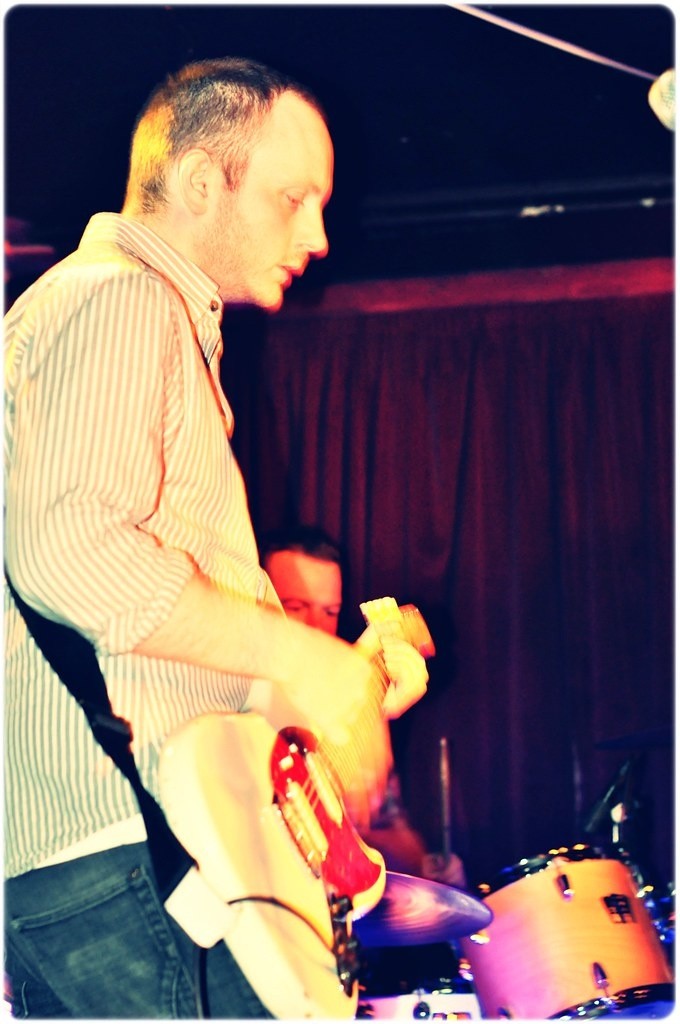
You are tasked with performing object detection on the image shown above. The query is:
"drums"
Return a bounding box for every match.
[458,841,676,1020]
[356,975,482,1021]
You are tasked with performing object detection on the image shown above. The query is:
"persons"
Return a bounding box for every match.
[260,526,469,992]
[3,55,430,1020]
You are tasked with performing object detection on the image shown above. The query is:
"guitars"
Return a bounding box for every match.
[154,592,438,1021]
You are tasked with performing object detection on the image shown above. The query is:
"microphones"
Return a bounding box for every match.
[586,762,632,835]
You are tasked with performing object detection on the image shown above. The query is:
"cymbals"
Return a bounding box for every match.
[348,869,496,951]
